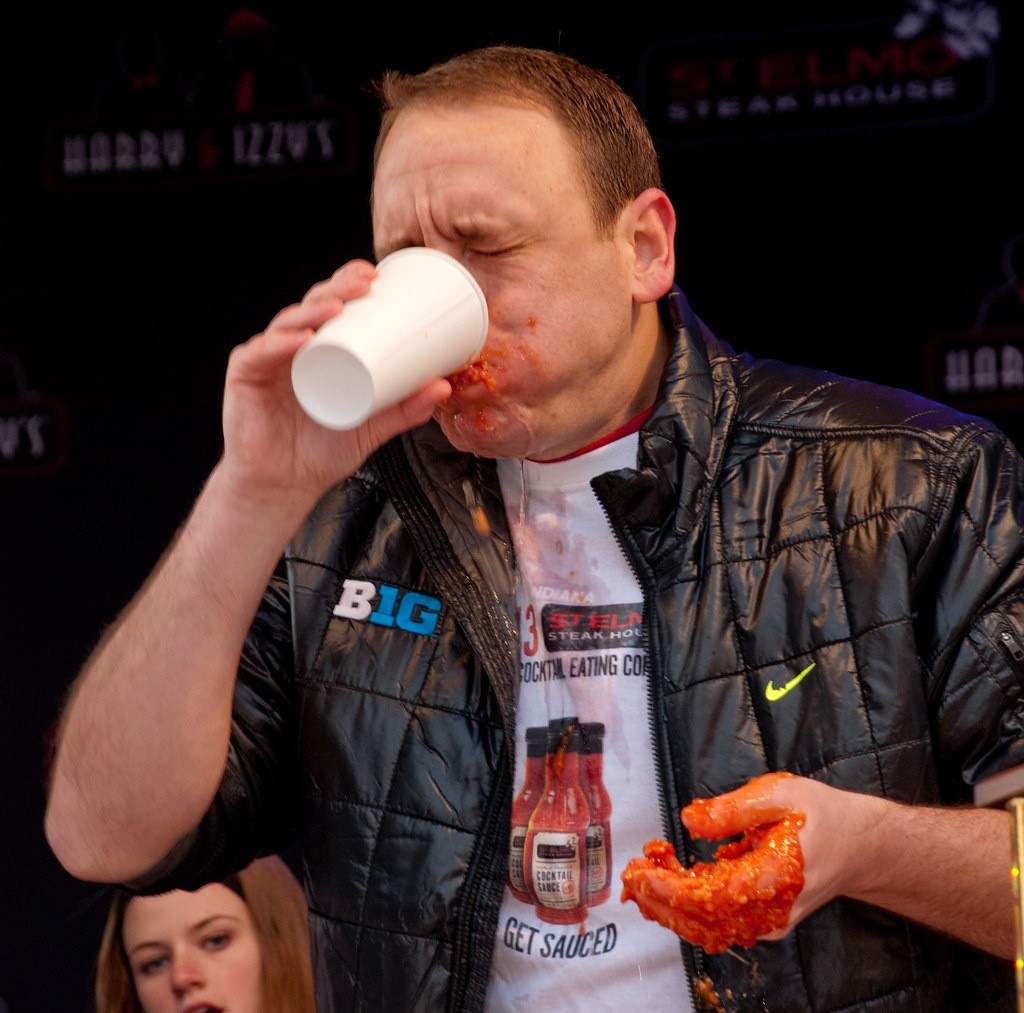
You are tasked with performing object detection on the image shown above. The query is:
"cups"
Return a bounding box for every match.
[290,241,490,433]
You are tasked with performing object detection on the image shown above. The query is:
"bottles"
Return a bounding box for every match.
[506,725,547,905]
[576,721,612,908]
[522,716,592,925]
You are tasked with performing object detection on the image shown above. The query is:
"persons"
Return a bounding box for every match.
[46,45,1024,1013]
[96,853,319,1013]
